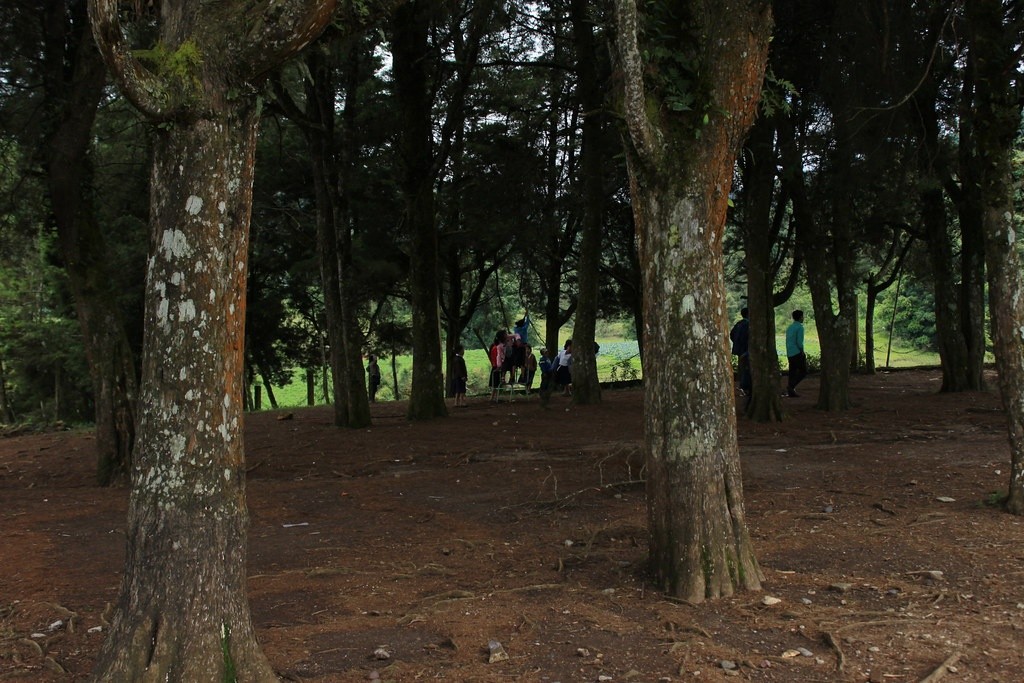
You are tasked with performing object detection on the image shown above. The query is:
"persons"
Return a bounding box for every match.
[730,307,751,396]
[538,340,571,405]
[366,356,380,408]
[489,309,537,403]
[452,345,469,408]
[786,310,808,397]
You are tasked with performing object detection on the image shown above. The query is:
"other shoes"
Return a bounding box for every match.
[460,405,468,408]
[455,406,460,407]
[788,390,800,397]
